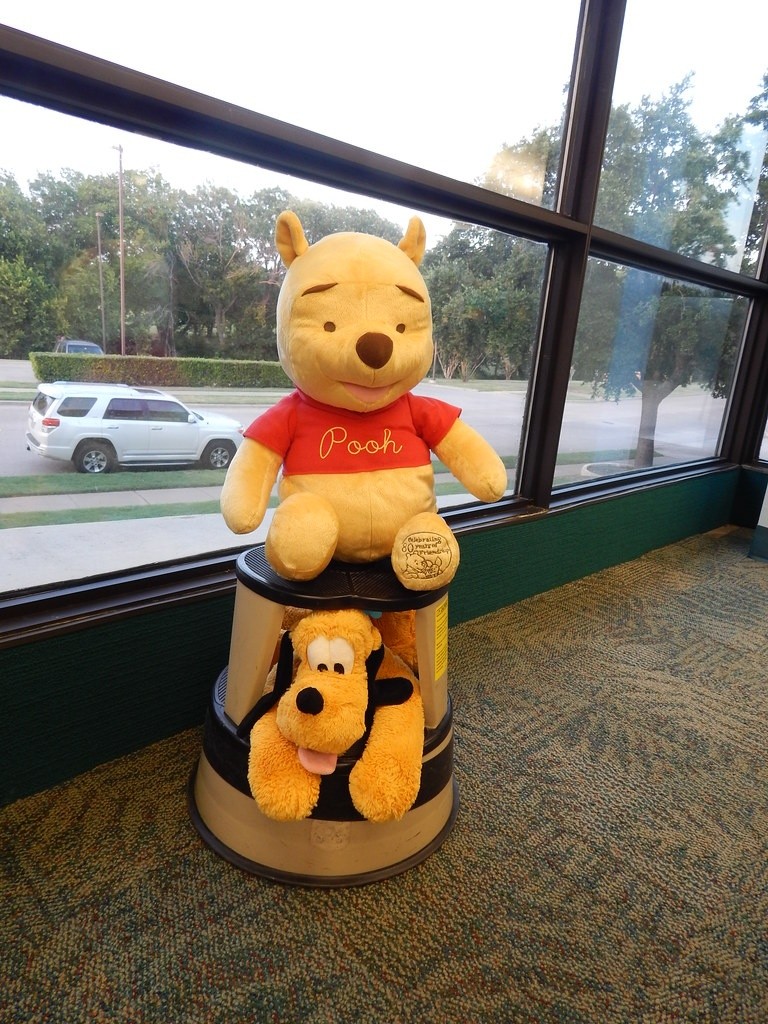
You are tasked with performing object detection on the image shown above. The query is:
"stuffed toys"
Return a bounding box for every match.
[237,609,423,823]
[218,210,509,592]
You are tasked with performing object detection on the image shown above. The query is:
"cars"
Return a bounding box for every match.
[54,381,129,387]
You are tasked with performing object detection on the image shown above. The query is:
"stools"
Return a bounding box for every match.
[186,663,460,890]
[224,542,450,730]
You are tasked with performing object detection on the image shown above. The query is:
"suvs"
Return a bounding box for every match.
[26,383,248,473]
[53,339,105,355]
